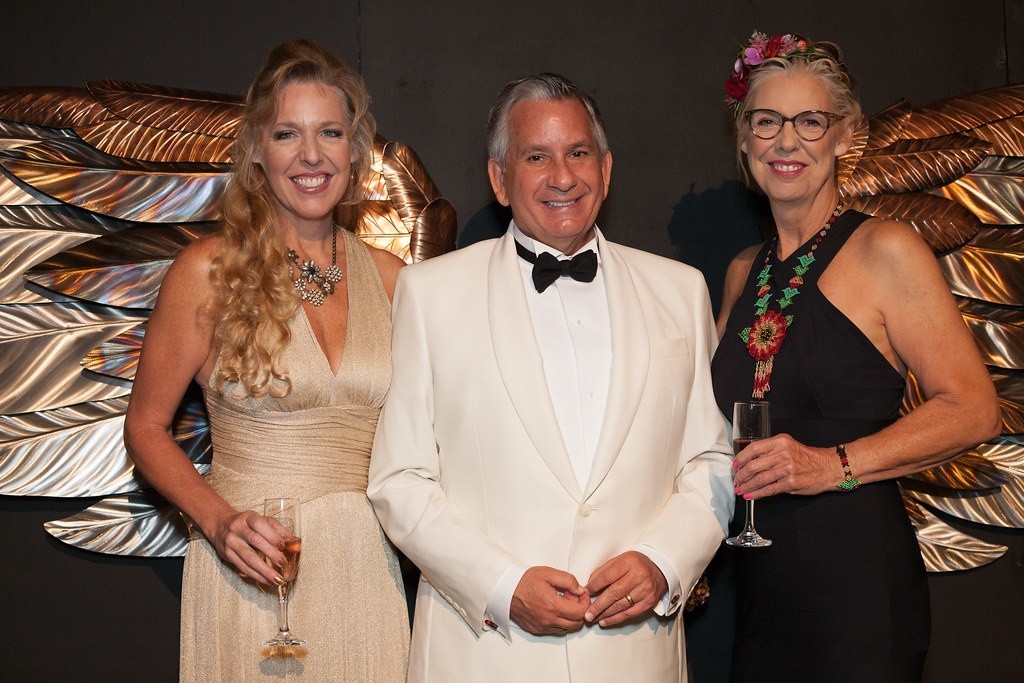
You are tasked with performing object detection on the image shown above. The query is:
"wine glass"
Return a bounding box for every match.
[262,497,309,647]
[726,401,772,547]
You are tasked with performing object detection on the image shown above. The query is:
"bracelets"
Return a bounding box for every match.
[836,443,862,492]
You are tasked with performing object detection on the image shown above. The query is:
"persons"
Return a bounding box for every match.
[684,28,1001,682]
[365,81,736,683]
[123,51,411,683]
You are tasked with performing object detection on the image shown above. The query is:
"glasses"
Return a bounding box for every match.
[743,109,845,141]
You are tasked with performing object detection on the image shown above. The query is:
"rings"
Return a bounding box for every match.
[625,594,634,606]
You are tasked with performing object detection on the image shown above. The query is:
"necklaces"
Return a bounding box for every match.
[273,220,343,307]
[738,199,844,408]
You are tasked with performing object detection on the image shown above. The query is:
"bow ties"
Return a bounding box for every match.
[514,239,598,293]
